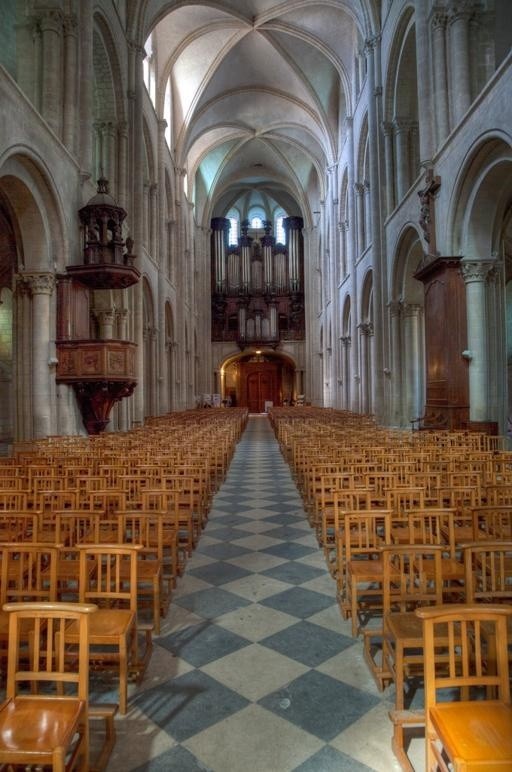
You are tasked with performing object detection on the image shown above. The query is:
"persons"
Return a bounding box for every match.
[418,180,434,243]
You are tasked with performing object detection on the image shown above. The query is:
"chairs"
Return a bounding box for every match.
[458,541,512,700]
[1,404,249,637]
[415,603,512,772]
[0,601,98,772]
[0,541,64,696]
[55,543,145,715]
[267,406,511,637]
[376,544,470,710]
